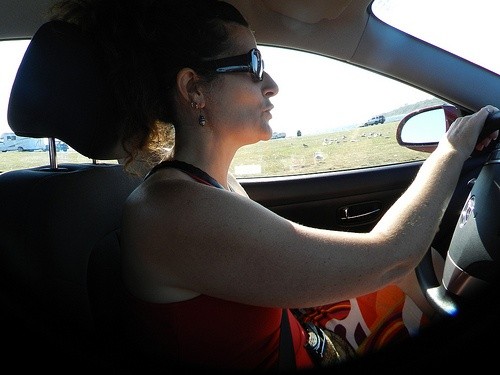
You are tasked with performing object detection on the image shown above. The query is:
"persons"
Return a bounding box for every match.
[57,0,500,375]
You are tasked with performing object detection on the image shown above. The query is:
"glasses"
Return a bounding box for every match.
[194,48,265,82]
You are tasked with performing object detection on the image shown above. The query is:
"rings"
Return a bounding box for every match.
[483,107,492,114]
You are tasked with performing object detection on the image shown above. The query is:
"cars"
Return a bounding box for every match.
[365,115,386,126]
[44,141,70,152]
[271,131,286,139]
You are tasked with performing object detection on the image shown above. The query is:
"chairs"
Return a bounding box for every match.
[0,20,150,375]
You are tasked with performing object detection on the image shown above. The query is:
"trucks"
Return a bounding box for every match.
[0,133,44,152]
